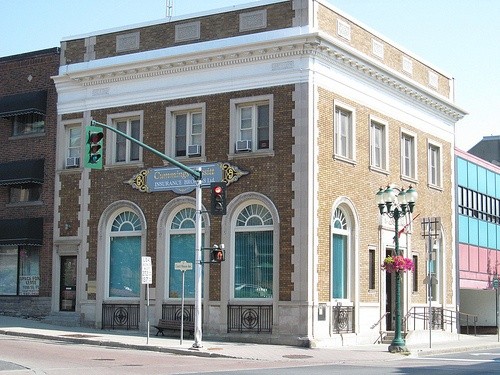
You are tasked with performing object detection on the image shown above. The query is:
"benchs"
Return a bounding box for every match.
[152,318,194,340]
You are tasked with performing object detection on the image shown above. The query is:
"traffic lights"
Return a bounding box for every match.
[84,125,103,169]
[212,249,225,261]
[210,182,227,215]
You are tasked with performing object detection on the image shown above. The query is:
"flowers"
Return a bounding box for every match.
[380,254,416,274]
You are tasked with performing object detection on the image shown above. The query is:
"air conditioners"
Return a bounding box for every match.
[236,139,251,151]
[66,156,80,167]
[187,144,201,155]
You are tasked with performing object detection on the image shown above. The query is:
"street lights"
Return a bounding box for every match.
[489,271,500,335]
[374,182,418,350]
